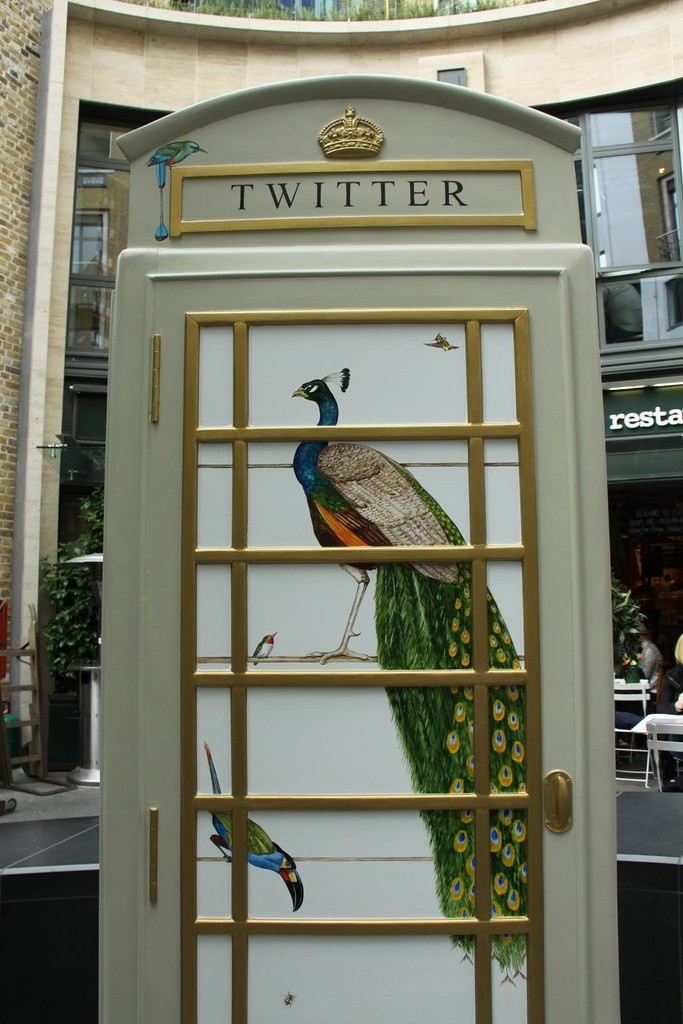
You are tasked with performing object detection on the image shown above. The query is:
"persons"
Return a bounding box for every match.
[614,626,663,730]
[655,633,683,786]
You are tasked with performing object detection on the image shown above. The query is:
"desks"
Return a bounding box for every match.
[631,713,683,788]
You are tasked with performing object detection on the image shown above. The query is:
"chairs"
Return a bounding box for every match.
[614,679,654,783]
[646,722,683,792]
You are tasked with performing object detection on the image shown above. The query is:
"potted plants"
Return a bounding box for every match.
[39,481,104,771]
